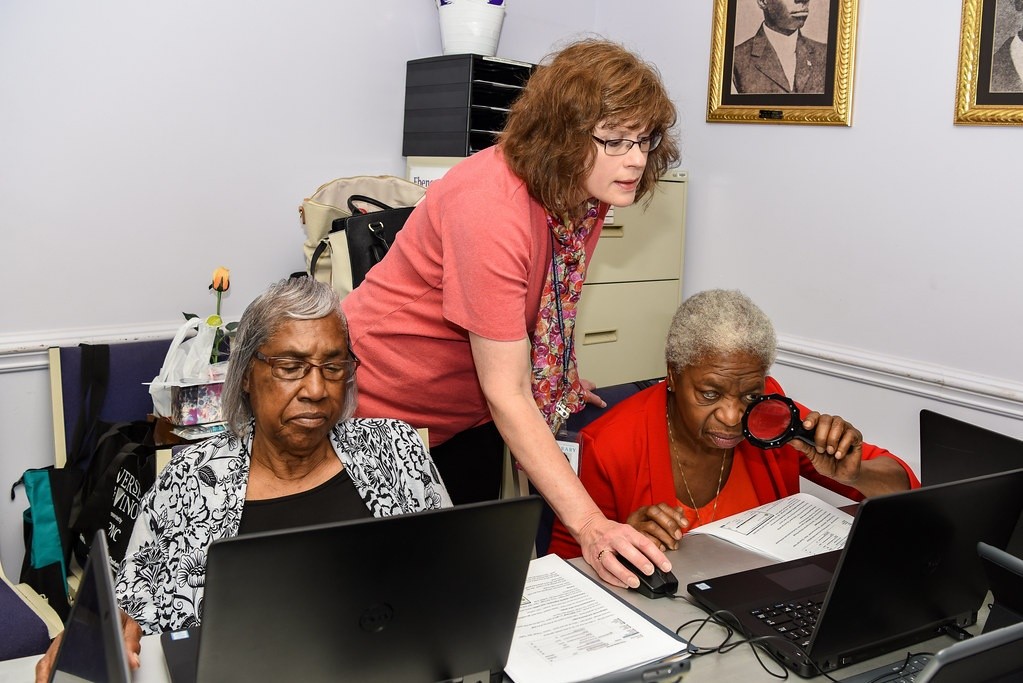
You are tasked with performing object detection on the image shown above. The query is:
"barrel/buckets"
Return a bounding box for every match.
[437,0,506,57]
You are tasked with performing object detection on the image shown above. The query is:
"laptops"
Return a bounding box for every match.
[159,495,545,683]
[685,469,1023,683]
[47,529,132,683]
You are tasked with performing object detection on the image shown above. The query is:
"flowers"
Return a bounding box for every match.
[183,265,240,362]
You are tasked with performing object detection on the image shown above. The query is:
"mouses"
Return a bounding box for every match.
[617,552,679,599]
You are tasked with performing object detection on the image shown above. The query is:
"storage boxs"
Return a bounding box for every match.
[170,384,225,427]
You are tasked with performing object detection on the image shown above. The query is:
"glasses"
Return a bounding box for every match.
[591,135,663,156]
[254,349,362,381]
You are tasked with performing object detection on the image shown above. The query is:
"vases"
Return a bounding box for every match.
[435,0,505,56]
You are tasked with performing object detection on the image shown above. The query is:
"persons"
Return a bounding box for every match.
[340,38,681,590]
[548,289,921,560]
[35,274,453,683]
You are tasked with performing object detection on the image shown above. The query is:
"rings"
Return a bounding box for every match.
[598,549,615,560]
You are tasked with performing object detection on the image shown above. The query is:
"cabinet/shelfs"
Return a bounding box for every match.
[404,156,688,389]
[402,53,540,157]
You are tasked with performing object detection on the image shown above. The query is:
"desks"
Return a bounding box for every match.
[516,504,993,683]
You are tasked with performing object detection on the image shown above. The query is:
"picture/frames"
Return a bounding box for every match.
[953,0,1023,127]
[705,0,859,127]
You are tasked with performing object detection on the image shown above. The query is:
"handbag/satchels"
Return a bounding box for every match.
[147,317,216,417]
[19,417,183,624]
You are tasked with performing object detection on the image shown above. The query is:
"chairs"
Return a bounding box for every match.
[526,377,667,560]
[47,337,430,607]
[0,560,65,662]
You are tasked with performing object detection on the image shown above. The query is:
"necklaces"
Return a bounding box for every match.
[667,400,726,526]
